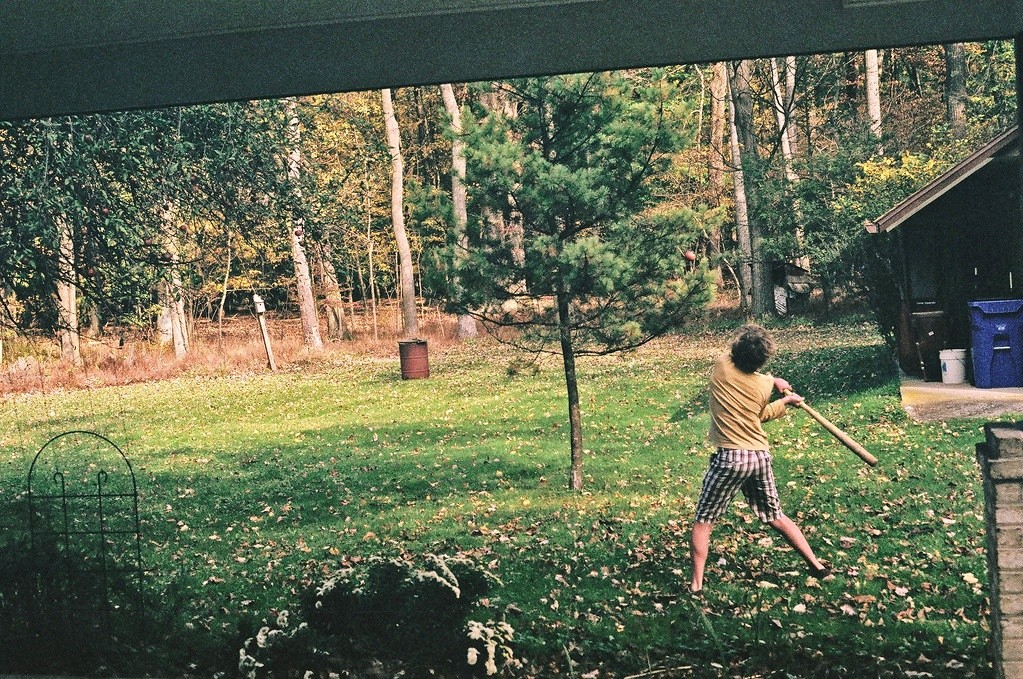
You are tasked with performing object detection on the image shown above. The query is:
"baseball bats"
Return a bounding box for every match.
[765,371,879,468]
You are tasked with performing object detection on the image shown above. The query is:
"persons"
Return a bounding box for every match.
[678,323,831,596]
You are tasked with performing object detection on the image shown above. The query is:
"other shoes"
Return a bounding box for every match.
[686,586,704,601]
[815,569,830,580]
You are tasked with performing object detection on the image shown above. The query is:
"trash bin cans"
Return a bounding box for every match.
[908,298,952,382]
[967,299,1023,389]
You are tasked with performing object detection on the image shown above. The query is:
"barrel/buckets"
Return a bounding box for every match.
[939,349,968,384]
[397,338,429,380]
